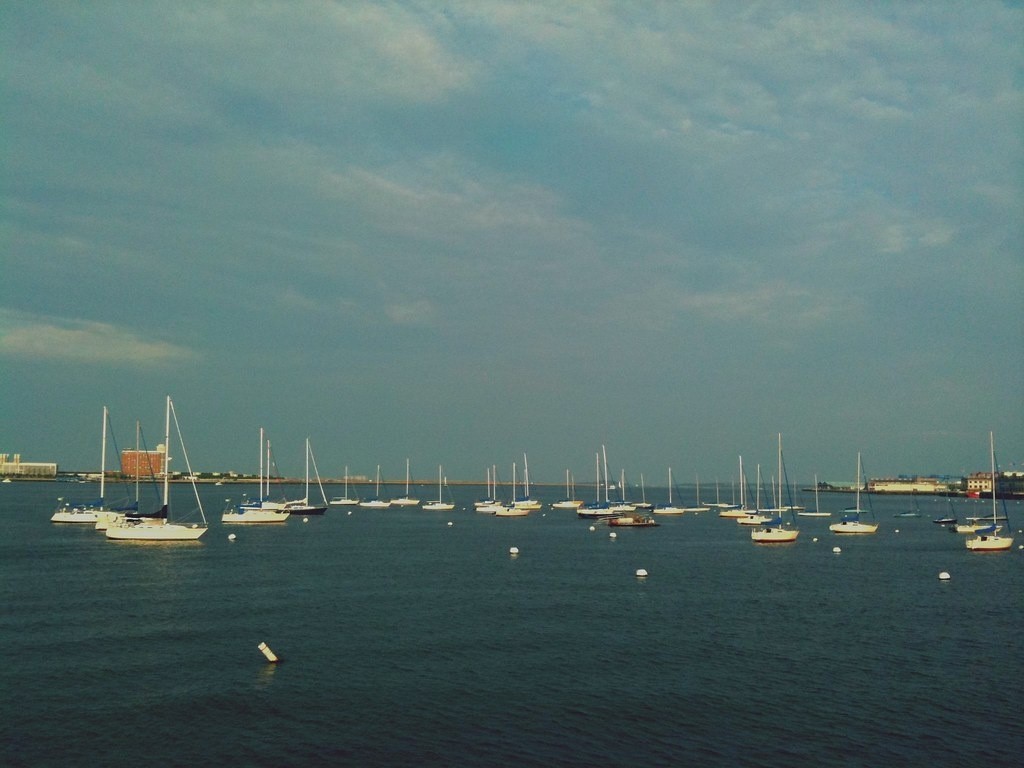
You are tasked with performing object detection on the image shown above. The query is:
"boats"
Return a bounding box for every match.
[965,514,1010,521]
[933,518,958,523]
[840,509,870,513]
[955,524,1002,534]
[896,511,922,518]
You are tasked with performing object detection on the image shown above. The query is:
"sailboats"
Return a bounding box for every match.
[54,398,213,540]
[704,432,808,542]
[390,459,421,505]
[328,465,363,507]
[360,465,392,507]
[552,468,584,508]
[965,432,1016,551]
[797,474,834,517]
[652,466,685,514]
[422,465,455,510]
[222,428,330,523]
[472,452,544,516]
[680,475,715,512]
[575,444,658,528]
[828,451,881,533]
[630,473,656,509]
